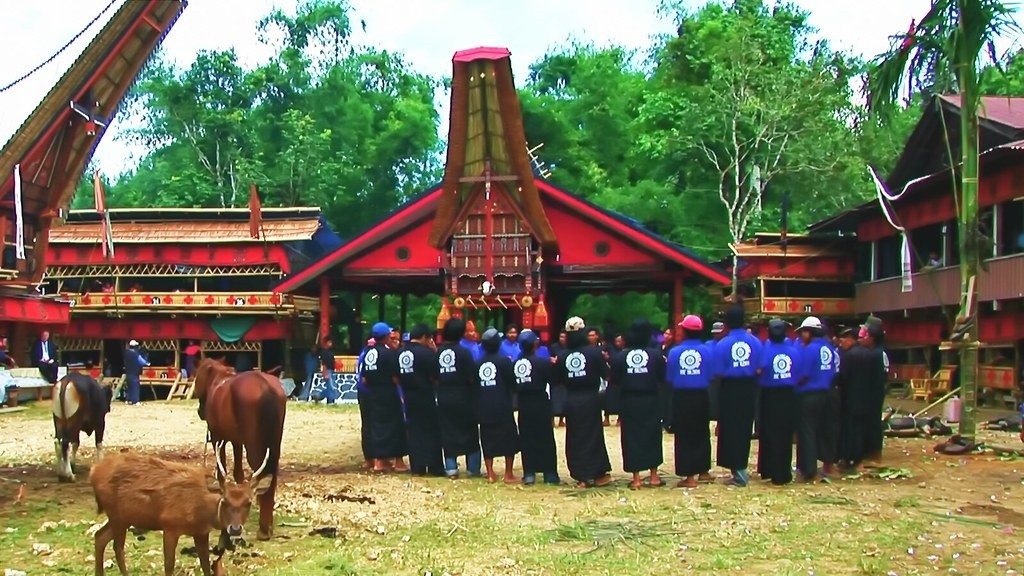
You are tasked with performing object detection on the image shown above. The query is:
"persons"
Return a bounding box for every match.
[32,330,59,384]
[356,303,890,488]
[122,340,151,404]
[0,335,15,411]
[923,248,945,269]
[298,337,338,405]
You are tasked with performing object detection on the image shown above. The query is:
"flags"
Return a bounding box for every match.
[93,172,105,213]
[249,186,261,240]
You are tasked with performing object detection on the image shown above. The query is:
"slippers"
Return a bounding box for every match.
[361,458,894,492]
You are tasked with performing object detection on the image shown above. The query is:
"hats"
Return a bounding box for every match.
[711,322,724,333]
[482,328,504,343]
[793,316,822,333]
[770,321,785,334]
[860,317,882,330]
[518,328,535,345]
[372,322,393,337]
[402,333,410,342]
[565,316,585,331]
[129,340,139,347]
[837,326,852,337]
[677,314,702,330]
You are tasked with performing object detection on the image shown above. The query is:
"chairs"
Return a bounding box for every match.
[910,370,951,403]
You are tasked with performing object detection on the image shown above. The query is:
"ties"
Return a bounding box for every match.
[43,341,47,352]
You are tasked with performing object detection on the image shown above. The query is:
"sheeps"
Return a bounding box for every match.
[88,440,272,576]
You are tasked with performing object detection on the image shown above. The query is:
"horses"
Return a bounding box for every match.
[192,355,287,541]
[51,373,116,481]
